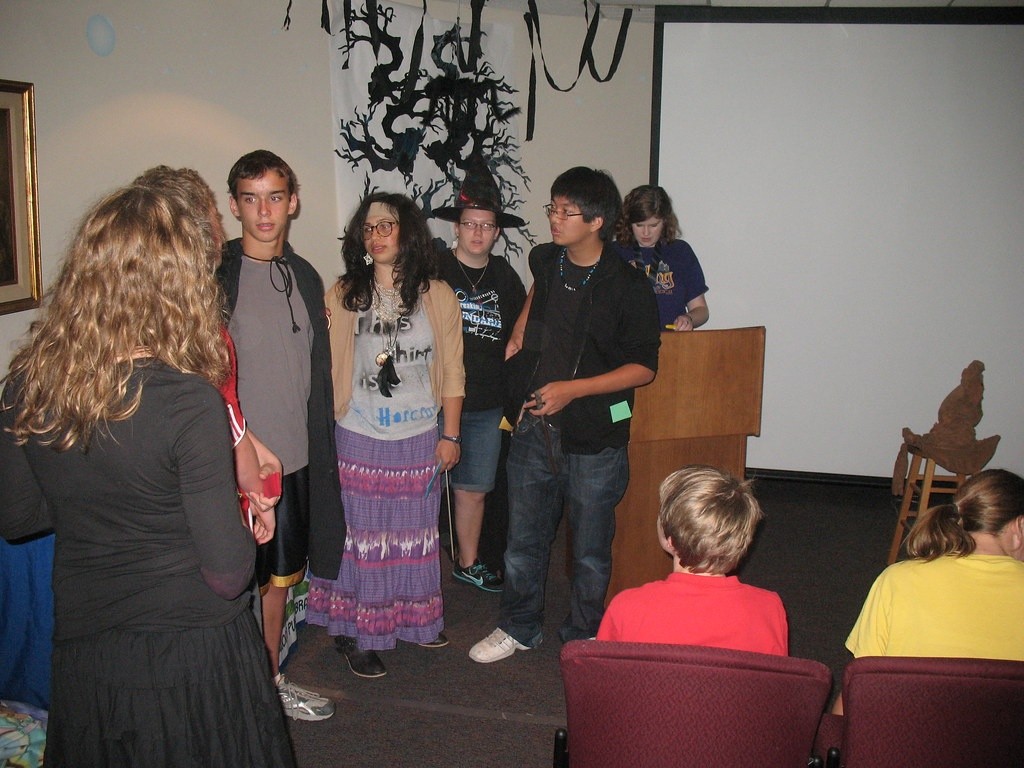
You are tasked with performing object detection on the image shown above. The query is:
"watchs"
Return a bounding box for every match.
[443,434,462,443]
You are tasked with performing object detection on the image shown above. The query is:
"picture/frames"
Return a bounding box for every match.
[0,79,44,316]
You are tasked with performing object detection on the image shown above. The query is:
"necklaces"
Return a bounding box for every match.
[373,283,401,368]
[560,246,600,292]
[455,247,488,294]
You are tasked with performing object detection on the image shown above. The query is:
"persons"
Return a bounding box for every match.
[468,160,661,664]
[595,462,788,657]
[0,183,296,767]
[834,468,1024,714]
[431,181,528,591]
[125,164,283,545]
[613,185,709,331]
[305,192,466,678]
[214,149,348,721]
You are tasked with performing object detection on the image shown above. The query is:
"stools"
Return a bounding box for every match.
[888,446,982,565]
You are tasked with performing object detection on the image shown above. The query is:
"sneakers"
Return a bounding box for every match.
[468,628,531,663]
[418,632,450,648]
[335,632,388,678]
[272,674,335,721]
[453,555,504,591]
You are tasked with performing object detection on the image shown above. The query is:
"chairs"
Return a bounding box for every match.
[551,638,1024,768]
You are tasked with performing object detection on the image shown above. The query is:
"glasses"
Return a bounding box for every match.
[458,221,497,232]
[363,220,399,240]
[542,203,584,220]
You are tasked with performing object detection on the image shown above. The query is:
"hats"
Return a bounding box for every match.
[431,153,527,229]
